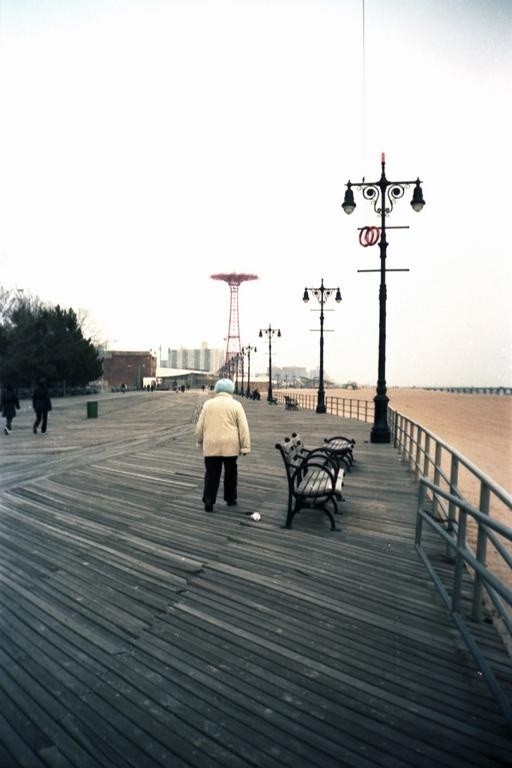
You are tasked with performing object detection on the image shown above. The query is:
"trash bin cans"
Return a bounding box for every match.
[86,401,98,418]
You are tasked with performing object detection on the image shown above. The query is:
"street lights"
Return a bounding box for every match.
[302,279,341,412]
[258,325,283,405]
[219,345,257,400]
[338,152,428,441]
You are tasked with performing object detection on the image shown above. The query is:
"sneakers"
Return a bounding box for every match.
[3,426,11,436]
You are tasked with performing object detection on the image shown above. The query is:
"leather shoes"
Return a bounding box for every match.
[205,497,214,513]
[226,499,238,506]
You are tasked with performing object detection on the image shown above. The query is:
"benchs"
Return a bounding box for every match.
[275,432,345,531]
[319,436,356,469]
[284,395,299,410]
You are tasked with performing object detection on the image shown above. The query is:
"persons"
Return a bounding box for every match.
[0,385,20,435]
[31,377,52,435]
[193,377,251,513]
